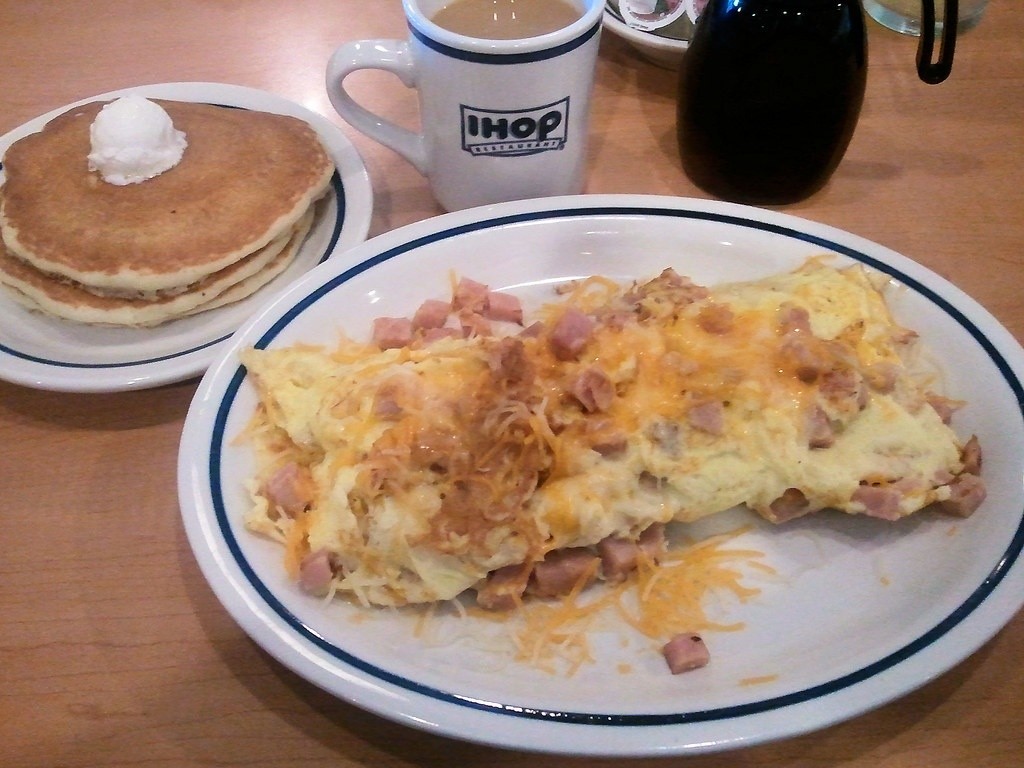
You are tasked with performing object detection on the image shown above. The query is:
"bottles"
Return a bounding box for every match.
[675,0,867,205]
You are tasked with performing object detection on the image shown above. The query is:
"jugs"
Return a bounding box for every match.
[862,0,989,86]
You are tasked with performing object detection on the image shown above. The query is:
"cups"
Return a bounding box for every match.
[325,0,605,212]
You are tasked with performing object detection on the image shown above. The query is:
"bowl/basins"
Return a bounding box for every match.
[604,7,690,70]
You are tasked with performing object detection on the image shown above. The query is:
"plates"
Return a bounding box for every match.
[0,83,373,393]
[177,191,1024,759]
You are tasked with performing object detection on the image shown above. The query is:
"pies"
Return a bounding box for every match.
[0,95,335,328]
